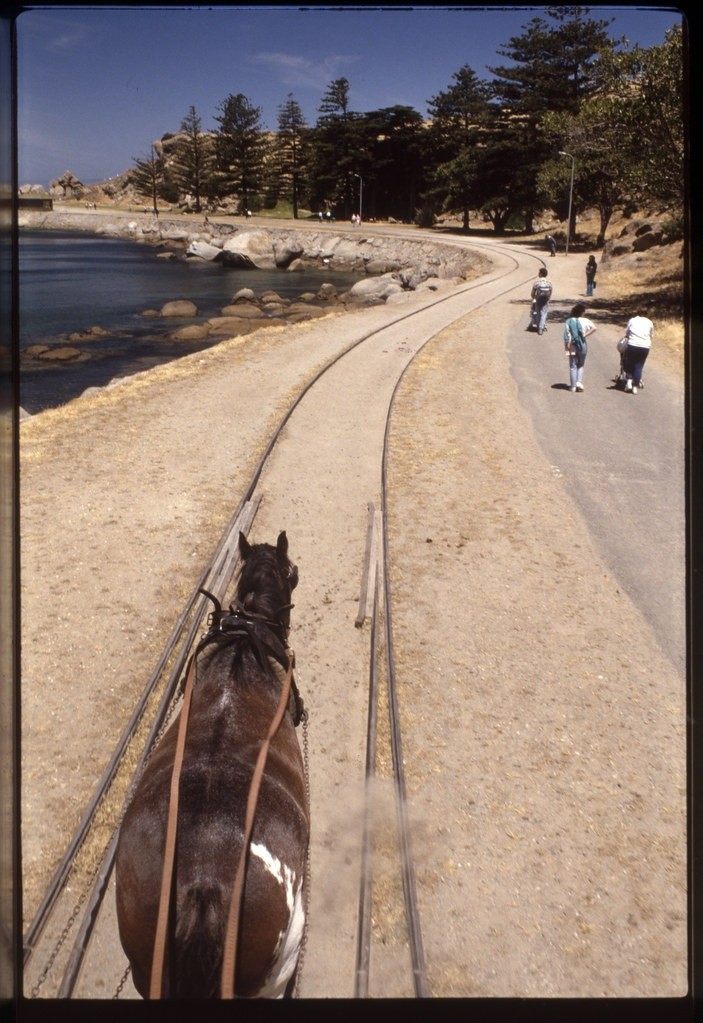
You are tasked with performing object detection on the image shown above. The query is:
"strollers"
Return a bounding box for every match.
[527,296,548,332]
[615,335,645,388]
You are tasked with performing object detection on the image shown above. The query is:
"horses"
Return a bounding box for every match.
[115,531,311,1000]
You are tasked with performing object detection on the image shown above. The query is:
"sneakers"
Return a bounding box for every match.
[568,386,576,391]
[576,382,584,392]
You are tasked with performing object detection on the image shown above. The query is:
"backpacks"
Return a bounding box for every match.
[537,286,552,305]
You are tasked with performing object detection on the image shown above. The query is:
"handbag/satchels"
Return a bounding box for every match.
[617,337,629,353]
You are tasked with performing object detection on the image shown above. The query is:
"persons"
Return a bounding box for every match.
[545,234,557,257]
[319,211,322,223]
[326,211,331,223]
[351,214,360,227]
[624,305,655,395]
[245,209,252,221]
[332,216,335,223]
[563,303,597,392]
[530,268,553,334]
[152,208,155,216]
[86,202,96,210]
[585,255,597,296]
[144,207,146,214]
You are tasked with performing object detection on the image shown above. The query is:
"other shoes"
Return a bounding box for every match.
[632,386,637,394]
[624,384,633,392]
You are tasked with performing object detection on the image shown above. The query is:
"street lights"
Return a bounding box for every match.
[355,173,362,223]
[558,150,574,253]
